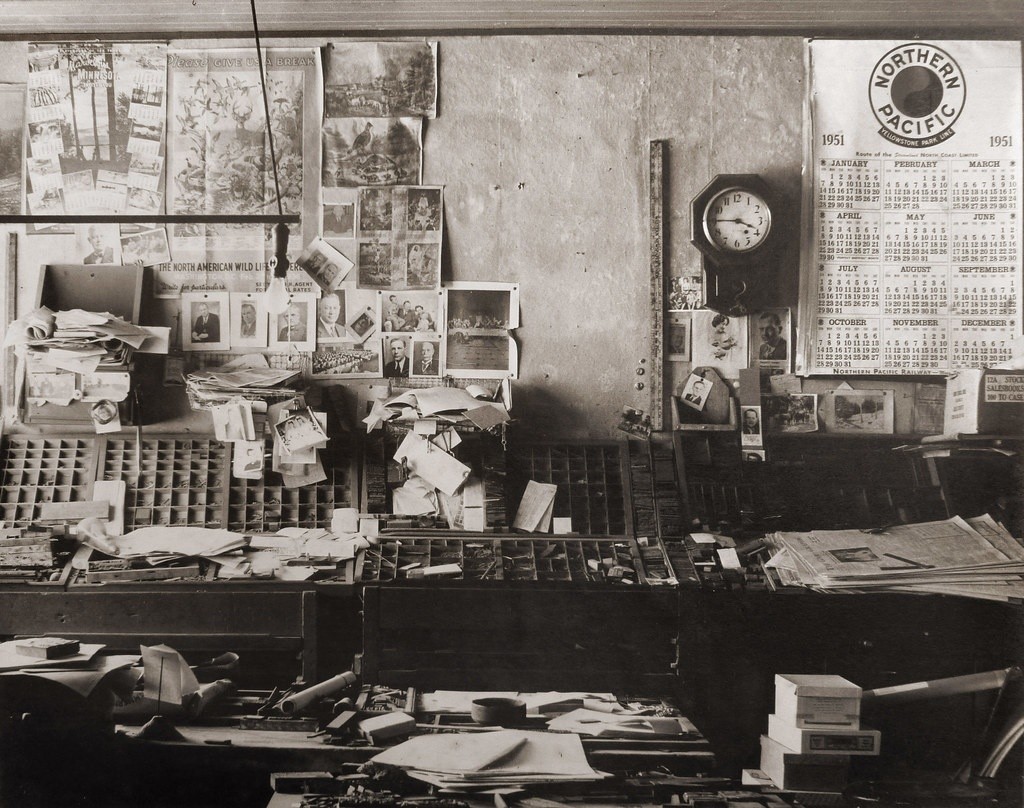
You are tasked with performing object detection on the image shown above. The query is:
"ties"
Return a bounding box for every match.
[395,361,401,373]
[331,327,336,336]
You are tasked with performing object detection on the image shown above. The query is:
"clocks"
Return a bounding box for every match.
[690,175,781,316]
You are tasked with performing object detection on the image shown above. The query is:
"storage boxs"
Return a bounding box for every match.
[759,674,879,793]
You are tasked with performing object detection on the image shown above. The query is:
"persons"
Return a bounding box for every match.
[759,312,787,360]
[743,409,761,435]
[95,407,112,419]
[240,303,256,338]
[413,340,439,375]
[311,349,374,373]
[670,328,684,354]
[282,416,323,451]
[668,278,698,310]
[684,382,705,406]
[303,253,325,276]
[242,448,260,470]
[383,337,408,377]
[191,303,220,342]
[123,235,166,261]
[317,294,346,337]
[384,293,435,331]
[315,263,338,291]
[280,304,307,342]
[707,315,731,360]
[83,224,114,265]
[326,206,352,233]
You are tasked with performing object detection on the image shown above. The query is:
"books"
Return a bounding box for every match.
[16,638,80,659]
